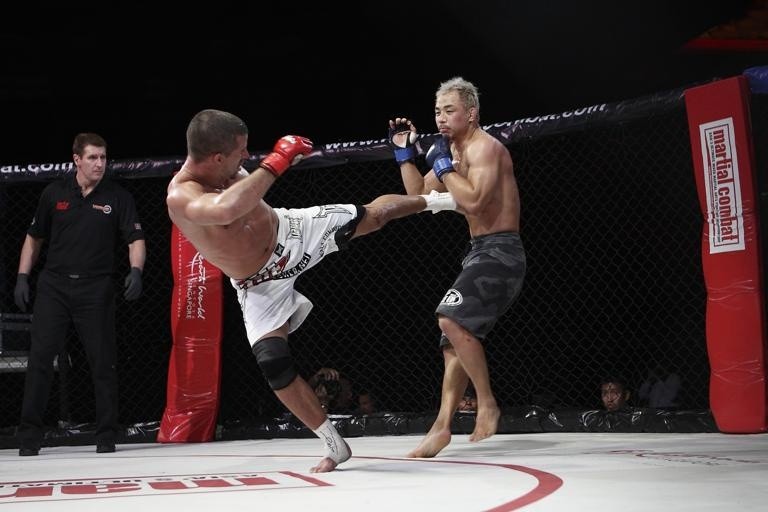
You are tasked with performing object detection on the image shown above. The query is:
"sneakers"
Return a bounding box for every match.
[95,437,117,454]
[18,440,40,457]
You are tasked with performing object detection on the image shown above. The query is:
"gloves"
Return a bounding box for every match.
[12,273,30,315]
[258,132,314,180]
[387,120,420,165]
[423,134,458,183]
[123,267,145,302]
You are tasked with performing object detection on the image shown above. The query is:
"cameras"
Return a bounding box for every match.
[318,377,338,399]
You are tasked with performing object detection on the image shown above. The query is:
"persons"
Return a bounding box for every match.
[388,78,526,459]
[166,109,467,474]
[13,133,146,456]
